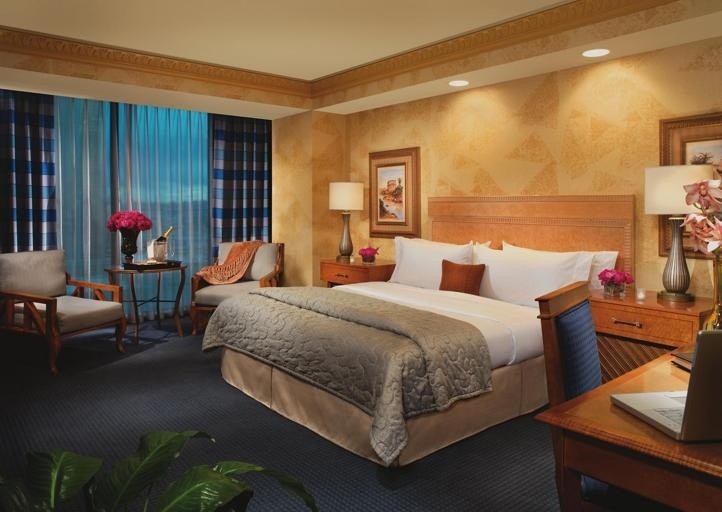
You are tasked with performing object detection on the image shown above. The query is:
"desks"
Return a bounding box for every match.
[101,259,191,347]
[533,327,722,512]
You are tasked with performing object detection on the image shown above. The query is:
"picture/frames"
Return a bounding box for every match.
[654,109,722,260]
[367,143,423,240]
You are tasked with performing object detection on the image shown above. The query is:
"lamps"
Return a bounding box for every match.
[327,178,367,258]
[641,163,717,302]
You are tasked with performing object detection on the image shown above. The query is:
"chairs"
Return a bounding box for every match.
[532,278,649,510]
[0,247,129,378]
[188,238,287,338]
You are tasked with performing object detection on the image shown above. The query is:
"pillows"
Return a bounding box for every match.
[478,248,598,305]
[439,258,487,296]
[393,234,493,283]
[382,229,475,296]
[498,236,624,296]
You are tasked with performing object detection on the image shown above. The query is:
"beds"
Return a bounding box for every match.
[204,189,637,472]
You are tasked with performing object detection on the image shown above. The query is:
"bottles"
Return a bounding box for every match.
[153,225,175,242]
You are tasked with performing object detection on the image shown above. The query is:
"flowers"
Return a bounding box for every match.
[678,178,722,256]
[106,208,152,234]
[671,170,722,272]
[357,246,381,256]
[596,268,635,285]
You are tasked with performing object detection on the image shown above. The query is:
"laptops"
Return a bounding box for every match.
[609,330,722,442]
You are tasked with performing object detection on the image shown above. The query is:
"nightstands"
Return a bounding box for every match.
[578,285,716,386]
[318,256,398,290]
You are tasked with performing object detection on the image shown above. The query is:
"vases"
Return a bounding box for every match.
[361,255,376,264]
[603,283,626,297]
[119,229,141,268]
[710,252,722,329]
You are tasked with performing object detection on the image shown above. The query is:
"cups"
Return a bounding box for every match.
[634,284,645,300]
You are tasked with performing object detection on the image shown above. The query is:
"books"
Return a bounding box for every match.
[670,351,694,372]
